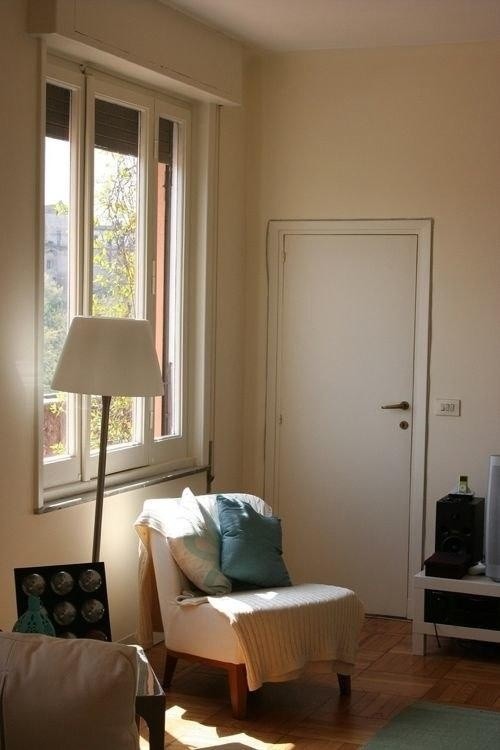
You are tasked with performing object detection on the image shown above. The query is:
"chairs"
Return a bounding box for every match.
[138,492,363,718]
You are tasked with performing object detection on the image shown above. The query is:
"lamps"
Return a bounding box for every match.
[52,312,164,564]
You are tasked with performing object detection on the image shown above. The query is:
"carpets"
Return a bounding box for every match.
[355,692,500,750]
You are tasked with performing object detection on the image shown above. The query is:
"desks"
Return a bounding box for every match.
[413,568,500,670]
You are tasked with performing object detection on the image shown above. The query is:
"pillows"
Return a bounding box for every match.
[164,485,293,597]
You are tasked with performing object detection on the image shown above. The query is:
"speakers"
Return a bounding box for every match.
[435,494,485,570]
